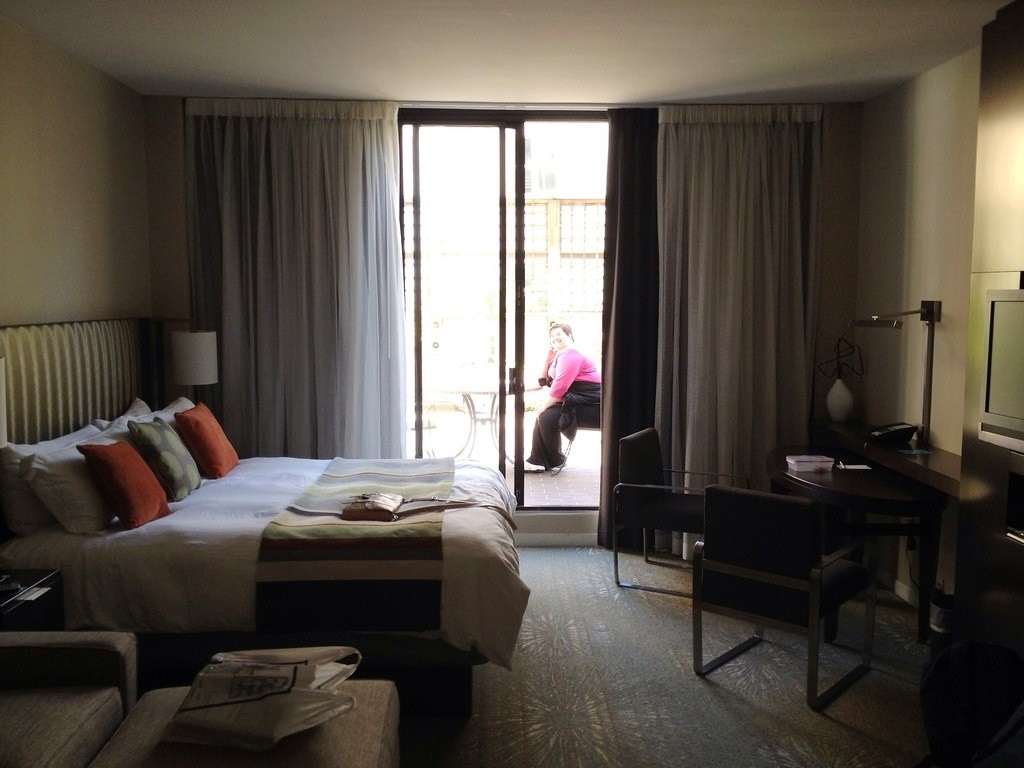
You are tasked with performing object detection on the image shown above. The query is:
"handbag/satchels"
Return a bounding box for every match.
[162,646,362,751]
[341,493,406,522]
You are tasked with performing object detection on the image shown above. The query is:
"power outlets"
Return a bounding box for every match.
[909,535,920,552]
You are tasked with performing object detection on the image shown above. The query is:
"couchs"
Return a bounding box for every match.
[0,631,137,768]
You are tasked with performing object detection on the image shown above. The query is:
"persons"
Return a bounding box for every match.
[524,323,601,473]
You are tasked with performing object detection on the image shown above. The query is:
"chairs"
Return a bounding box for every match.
[406,402,601,477]
[611,428,747,600]
[694,484,881,711]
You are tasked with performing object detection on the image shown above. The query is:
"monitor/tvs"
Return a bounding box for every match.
[977,290,1024,454]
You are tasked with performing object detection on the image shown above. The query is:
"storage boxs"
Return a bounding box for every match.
[786,455,834,472]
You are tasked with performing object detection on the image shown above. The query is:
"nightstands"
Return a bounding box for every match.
[0,567,66,634]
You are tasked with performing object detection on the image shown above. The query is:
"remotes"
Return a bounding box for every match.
[0,583,20,593]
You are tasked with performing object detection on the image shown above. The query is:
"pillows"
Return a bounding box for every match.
[74,441,171,529]
[19,415,131,535]
[4,423,103,535]
[92,398,152,432]
[127,417,201,502]
[130,397,195,430]
[174,400,239,479]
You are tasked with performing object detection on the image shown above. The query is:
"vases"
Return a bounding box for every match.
[826,378,854,422]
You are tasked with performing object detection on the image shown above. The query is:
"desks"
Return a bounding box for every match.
[769,444,946,645]
[440,385,542,464]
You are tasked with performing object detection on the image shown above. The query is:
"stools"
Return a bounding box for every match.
[85,680,398,768]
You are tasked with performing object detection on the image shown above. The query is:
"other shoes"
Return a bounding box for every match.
[524,461,545,473]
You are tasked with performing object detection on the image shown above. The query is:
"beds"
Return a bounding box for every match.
[0,319,530,722]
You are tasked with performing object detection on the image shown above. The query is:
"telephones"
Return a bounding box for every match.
[865,422,917,445]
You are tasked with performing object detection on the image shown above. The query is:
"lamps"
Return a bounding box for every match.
[848,300,942,444]
[171,329,217,405]
